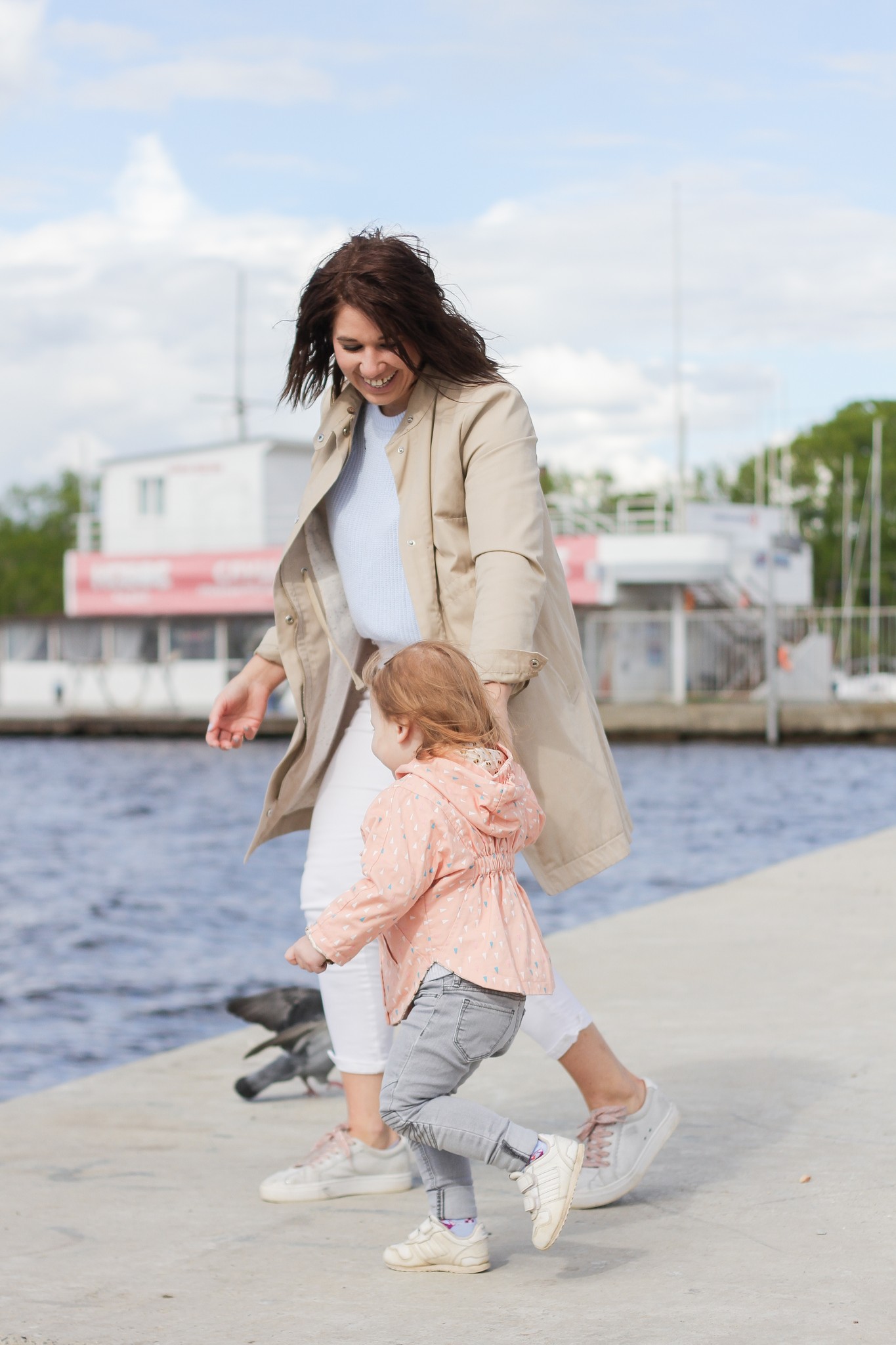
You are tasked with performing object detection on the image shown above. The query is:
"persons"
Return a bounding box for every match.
[205,225,683,1207]
[284,639,585,1274]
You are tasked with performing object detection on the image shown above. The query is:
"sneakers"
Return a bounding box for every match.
[571,1079,681,1208]
[261,1126,411,1201]
[509,1133,586,1251]
[383,1214,489,1275]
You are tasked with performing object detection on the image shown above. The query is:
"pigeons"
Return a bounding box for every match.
[225,986,342,1103]
